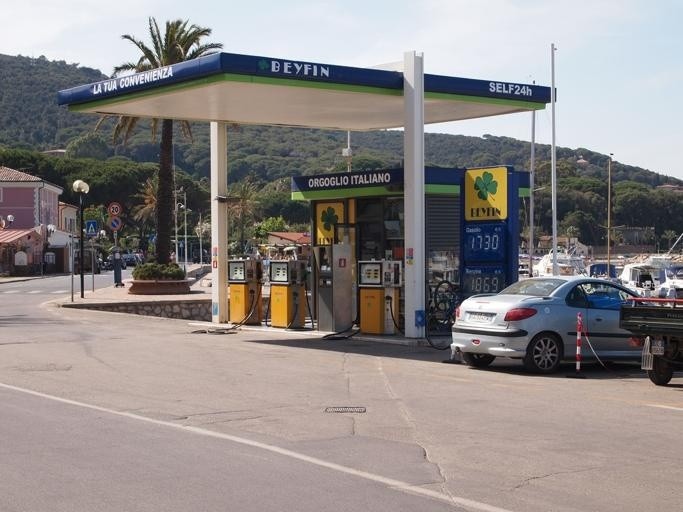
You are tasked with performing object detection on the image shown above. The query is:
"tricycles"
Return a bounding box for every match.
[617,305,683,386]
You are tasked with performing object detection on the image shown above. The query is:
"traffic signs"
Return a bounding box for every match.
[108,202,121,216]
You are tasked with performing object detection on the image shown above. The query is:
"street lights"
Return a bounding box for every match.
[72,179,89,298]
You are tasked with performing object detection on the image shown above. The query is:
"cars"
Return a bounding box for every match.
[193,250,211,264]
[101,252,144,271]
[451,274,655,375]
[537,251,682,298]
